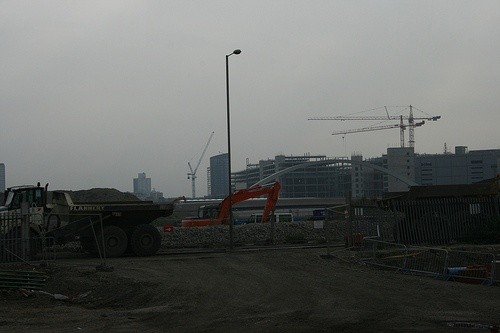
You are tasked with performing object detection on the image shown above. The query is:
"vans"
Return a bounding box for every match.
[244,211,294,222]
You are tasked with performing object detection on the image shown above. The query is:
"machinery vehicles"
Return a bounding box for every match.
[155,196,186,217]
[181,178,281,224]
[0,179,176,260]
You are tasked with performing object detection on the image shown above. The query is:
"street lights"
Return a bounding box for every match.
[225,48,244,250]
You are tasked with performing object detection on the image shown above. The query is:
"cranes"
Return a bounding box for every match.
[307,97,441,156]
[186,129,215,198]
